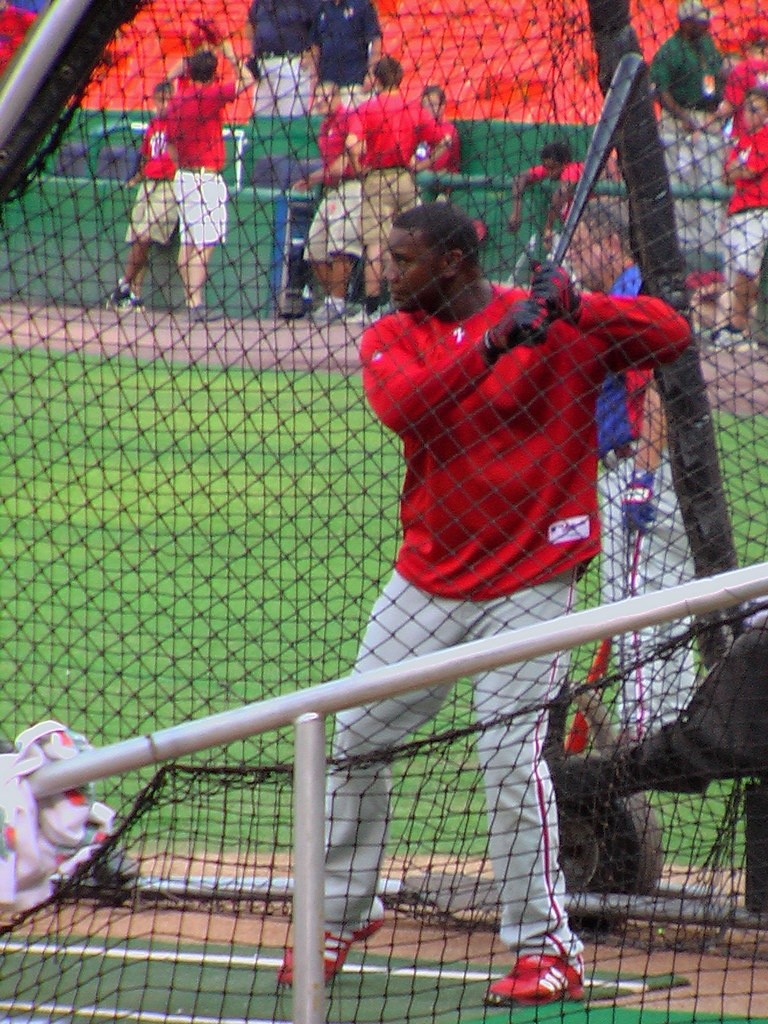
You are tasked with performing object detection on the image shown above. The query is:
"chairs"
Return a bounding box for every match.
[94,146,140,176]
[290,157,321,185]
[252,157,289,188]
[53,139,90,177]
[0,0,768,123]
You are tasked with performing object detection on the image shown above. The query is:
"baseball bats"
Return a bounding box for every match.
[568,639,613,754]
[518,51,646,343]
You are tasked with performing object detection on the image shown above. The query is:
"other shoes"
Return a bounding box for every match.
[348,303,391,325]
[106,280,145,313]
[310,299,347,320]
[697,325,762,355]
[186,305,228,321]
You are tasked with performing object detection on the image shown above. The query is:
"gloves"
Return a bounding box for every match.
[620,468,655,533]
[484,296,550,353]
[528,260,582,325]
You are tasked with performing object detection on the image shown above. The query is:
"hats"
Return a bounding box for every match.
[678,0,717,23]
[744,26,768,44]
[744,80,768,96]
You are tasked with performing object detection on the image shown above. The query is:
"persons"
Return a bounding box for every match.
[105,38,255,321]
[275,206,693,1005]
[312,0,383,115]
[288,61,460,325]
[245,0,322,118]
[506,143,583,253]
[644,1,767,351]
[569,203,691,741]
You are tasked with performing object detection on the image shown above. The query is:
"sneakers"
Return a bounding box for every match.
[275,894,386,987]
[479,951,587,1009]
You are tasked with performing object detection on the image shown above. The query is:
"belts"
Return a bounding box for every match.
[254,46,301,59]
[375,163,407,173]
[180,168,214,174]
